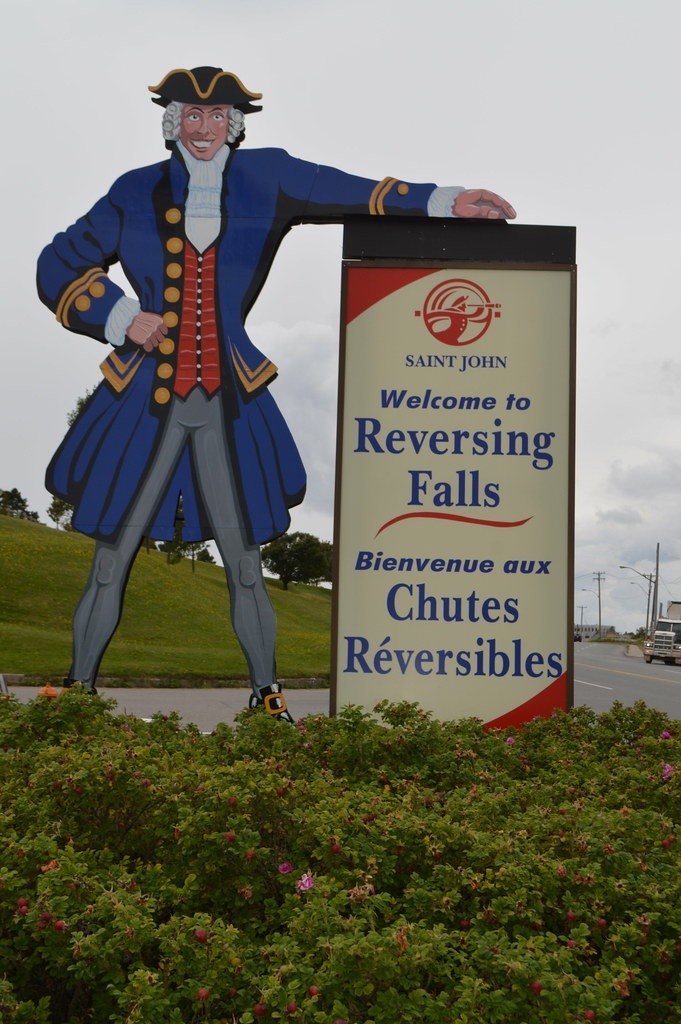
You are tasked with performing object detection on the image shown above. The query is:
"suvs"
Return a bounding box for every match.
[640,618,681,665]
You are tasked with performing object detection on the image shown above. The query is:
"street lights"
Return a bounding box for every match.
[618,564,657,642]
[576,603,588,642]
[582,586,601,641]
